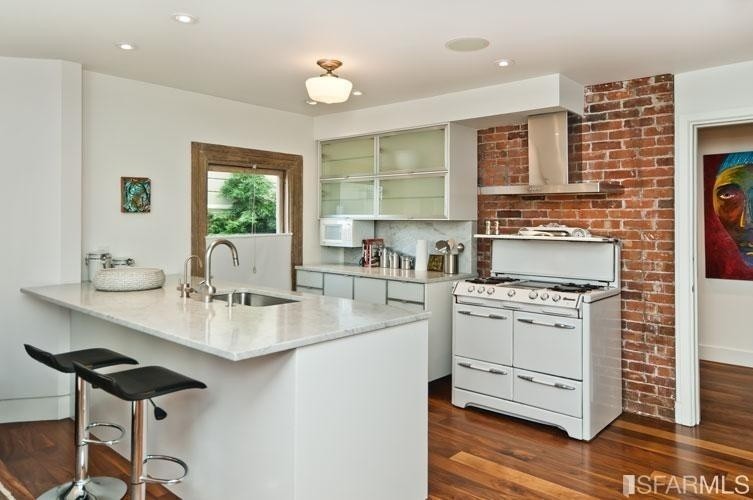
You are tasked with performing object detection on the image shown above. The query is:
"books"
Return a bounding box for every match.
[368,244,385,267]
[362,238,383,267]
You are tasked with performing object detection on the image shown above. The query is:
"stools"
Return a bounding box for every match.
[21,343,208,500]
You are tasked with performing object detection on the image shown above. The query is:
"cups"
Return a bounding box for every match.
[442,254,458,274]
[377,248,414,270]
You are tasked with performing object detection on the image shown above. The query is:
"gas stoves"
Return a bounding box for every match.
[453,275,612,319]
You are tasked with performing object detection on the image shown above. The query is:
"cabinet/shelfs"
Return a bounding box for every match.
[316,123,478,220]
[294,270,424,310]
[452,304,583,440]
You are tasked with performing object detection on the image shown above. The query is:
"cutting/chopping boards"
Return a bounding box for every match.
[476,111,624,196]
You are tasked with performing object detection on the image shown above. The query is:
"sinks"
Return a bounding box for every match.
[208,288,306,308]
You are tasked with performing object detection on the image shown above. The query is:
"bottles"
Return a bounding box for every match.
[492,220,500,235]
[485,220,492,235]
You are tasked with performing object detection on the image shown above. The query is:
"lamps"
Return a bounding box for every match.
[305,59,354,105]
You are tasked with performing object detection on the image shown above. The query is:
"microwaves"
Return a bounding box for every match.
[318,218,375,248]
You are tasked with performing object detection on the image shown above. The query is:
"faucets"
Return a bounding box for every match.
[181,255,203,298]
[205,302,217,344]
[183,298,190,312]
[203,238,239,302]
